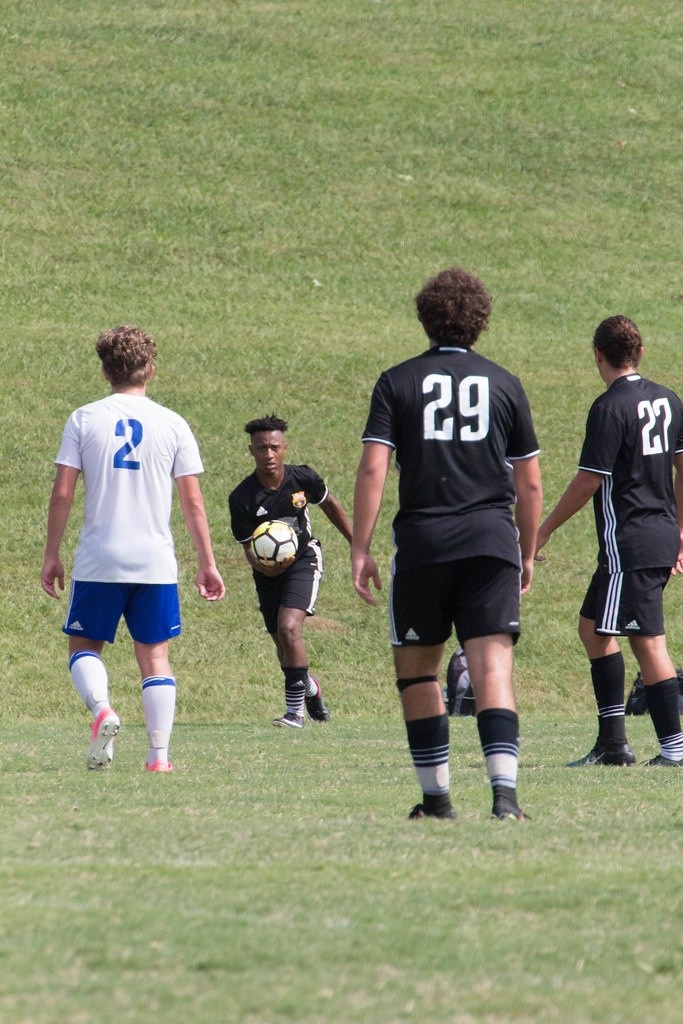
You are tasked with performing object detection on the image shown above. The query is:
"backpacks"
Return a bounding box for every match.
[443,648,478,717]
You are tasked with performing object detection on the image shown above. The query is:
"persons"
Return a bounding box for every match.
[533,315,683,767]
[40,324,226,773]
[228,411,353,730]
[350,265,543,820]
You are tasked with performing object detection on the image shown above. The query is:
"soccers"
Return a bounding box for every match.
[250,519,298,566]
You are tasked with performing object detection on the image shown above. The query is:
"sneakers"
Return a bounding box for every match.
[88,707,120,772]
[272,712,306,729]
[565,742,636,767]
[306,677,330,724]
[408,803,457,821]
[644,754,683,767]
[145,762,173,773]
[490,803,531,821]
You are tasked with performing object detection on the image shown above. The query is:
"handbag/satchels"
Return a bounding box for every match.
[624,670,683,716]
